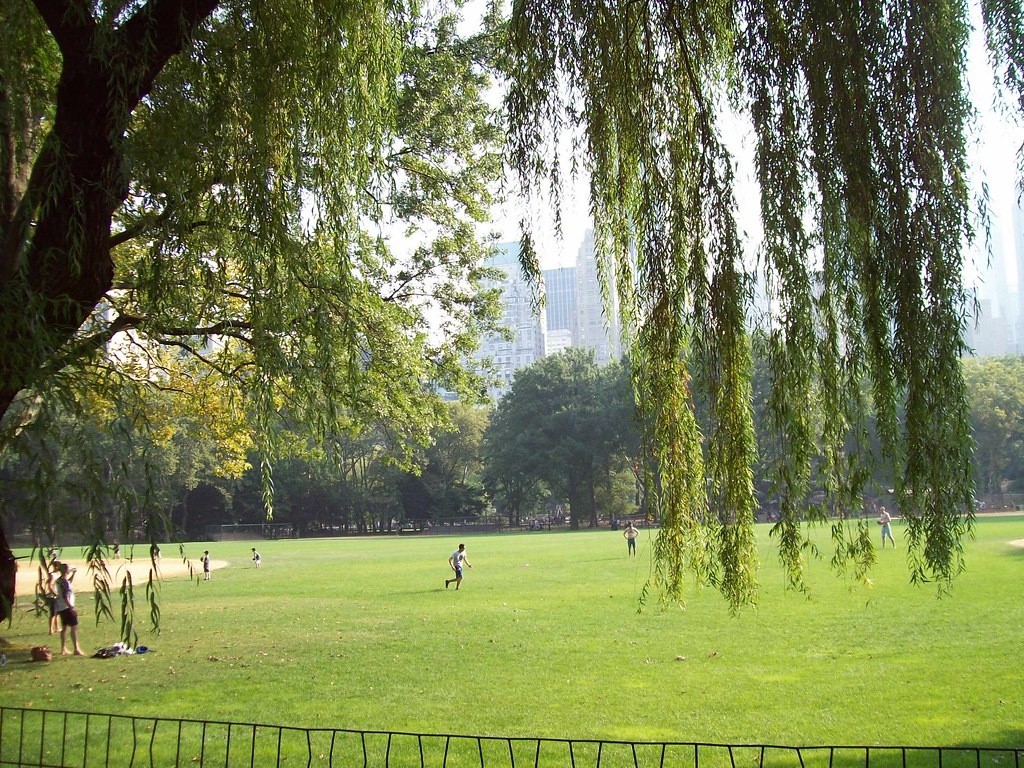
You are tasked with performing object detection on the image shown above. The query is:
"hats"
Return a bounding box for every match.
[137,646,149,654]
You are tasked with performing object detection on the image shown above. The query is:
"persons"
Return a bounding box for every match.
[56,563,86,656]
[623,522,639,557]
[46,561,63,634]
[878,507,896,549]
[150,542,161,576]
[200,551,210,580]
[523,510,563,531]
[112,538,121,559]
[251,548,261,568]
[445,543,472,591]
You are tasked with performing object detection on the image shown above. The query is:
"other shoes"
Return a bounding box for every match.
[446,580,449,588]
